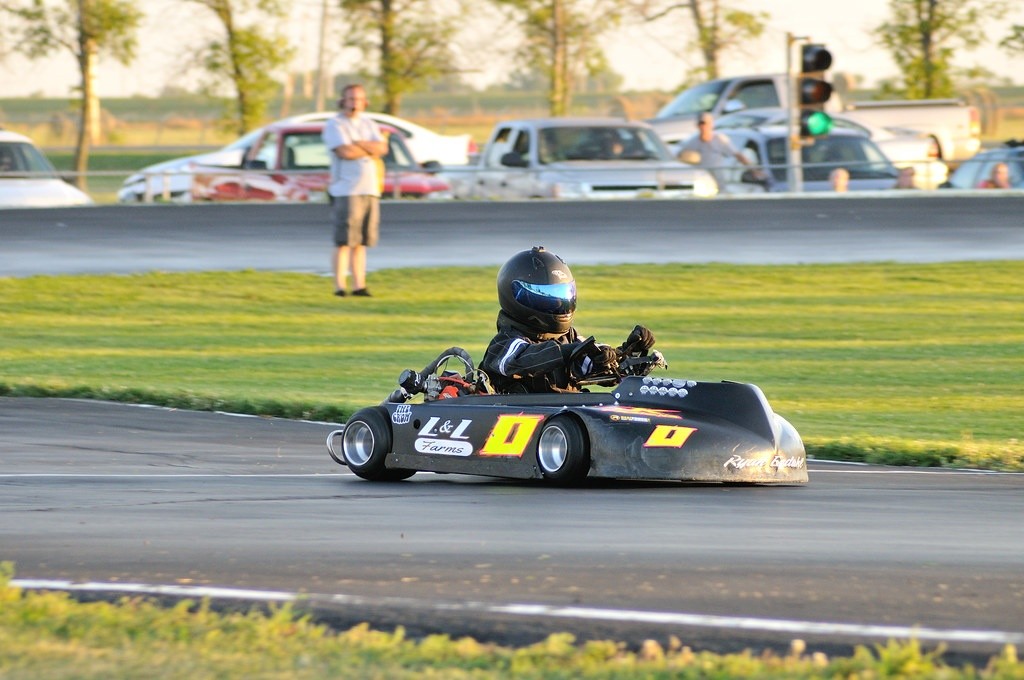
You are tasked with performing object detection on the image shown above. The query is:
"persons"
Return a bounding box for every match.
[678,113,755,192]
[983,164,1009,188]
[476,246,655,394]
[322,86,389,297]
[830,168,849,191]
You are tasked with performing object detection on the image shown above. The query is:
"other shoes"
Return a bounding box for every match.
[334,290,378,299]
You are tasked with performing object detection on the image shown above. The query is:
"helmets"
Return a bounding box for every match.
[498,245,578,338]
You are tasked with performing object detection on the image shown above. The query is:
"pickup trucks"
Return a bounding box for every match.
[651,74,982,194]
[194,123,452,205]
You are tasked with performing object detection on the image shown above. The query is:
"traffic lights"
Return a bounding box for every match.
[799,42,833,138]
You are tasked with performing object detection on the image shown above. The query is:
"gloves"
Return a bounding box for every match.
[625,323,658,352]
[594,344,617,368]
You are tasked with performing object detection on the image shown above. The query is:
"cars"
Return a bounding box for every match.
[947,141,1024,191]
[1,129,99,205]
[121,110,475,197]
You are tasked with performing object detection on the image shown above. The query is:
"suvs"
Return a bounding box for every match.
[478,118,720,197]
